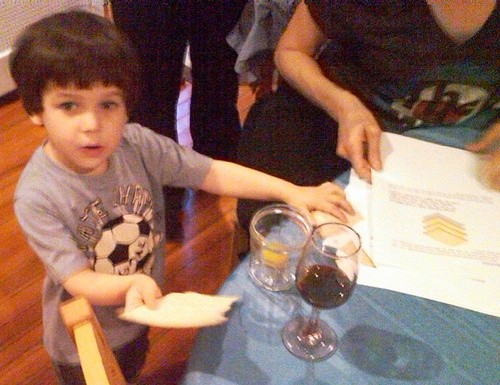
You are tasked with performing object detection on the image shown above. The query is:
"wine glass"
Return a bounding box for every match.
[281,222,362,361]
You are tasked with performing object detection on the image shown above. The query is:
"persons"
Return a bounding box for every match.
[109,0,250,208]
[7,9,355,385]
[271,0,500,193]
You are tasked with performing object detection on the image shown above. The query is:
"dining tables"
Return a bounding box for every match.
[187,125,500,385]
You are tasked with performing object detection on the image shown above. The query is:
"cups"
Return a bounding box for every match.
[248,204,312,292]
